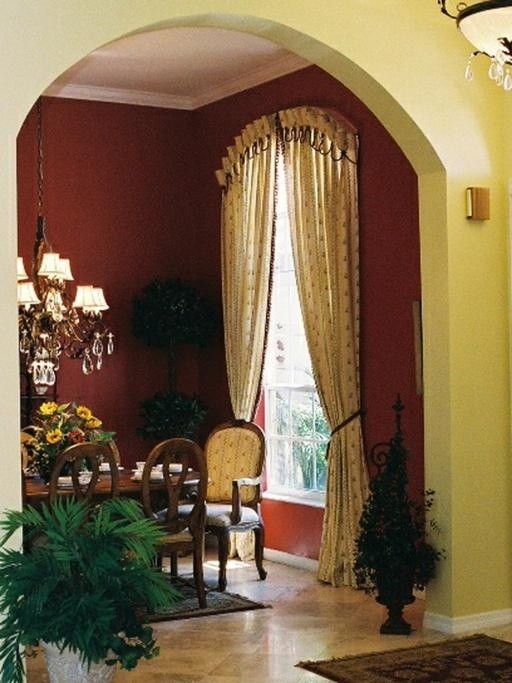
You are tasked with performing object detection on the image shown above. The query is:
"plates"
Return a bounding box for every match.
[46,461,194,491]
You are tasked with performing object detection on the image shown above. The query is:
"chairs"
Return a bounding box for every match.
[135,437,208,610]
[21,426,49,480]
[154,418,269,593]
[48,441,121,518]
[101,431,121,471]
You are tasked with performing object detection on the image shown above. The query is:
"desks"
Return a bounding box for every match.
[23,463,213,503]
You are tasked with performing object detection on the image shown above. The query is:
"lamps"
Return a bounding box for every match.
[431,0,511,94]
[15,90,120,396]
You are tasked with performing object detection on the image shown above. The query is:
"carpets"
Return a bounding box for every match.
[133,567,268,629]
[293,625,510,681]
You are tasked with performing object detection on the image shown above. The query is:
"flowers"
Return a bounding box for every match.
[20,398,118,481]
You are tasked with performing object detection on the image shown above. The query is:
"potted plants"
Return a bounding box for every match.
[0,482,183,683]
[349,431,445,635]
